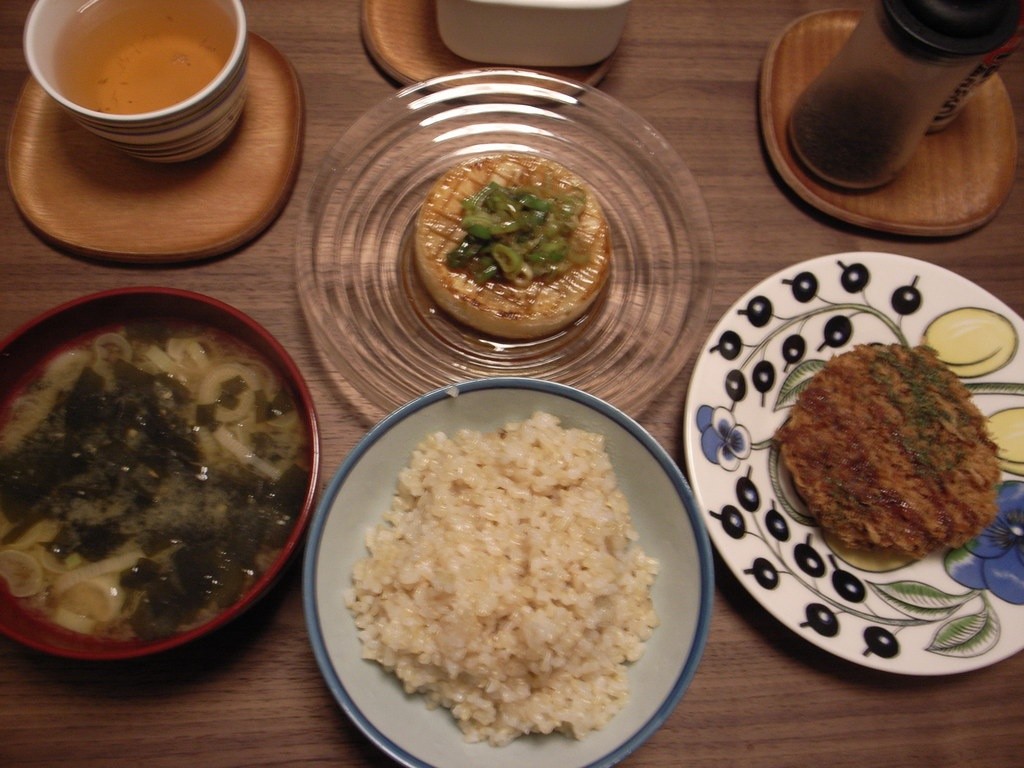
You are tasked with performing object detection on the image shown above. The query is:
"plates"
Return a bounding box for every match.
[293,70,715,433]
[685,251,1024,676]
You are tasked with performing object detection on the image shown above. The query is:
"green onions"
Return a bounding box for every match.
[0,332,298,635]
[447,179,591,286]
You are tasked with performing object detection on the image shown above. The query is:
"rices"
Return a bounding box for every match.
[347,410,660,747]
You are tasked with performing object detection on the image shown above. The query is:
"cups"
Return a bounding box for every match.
[435,1,628,68]
[786,0,1024,191]
[23,1,248,162]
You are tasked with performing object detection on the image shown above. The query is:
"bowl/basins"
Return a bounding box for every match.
[0,286,323,662]
[304,379,718,768]
